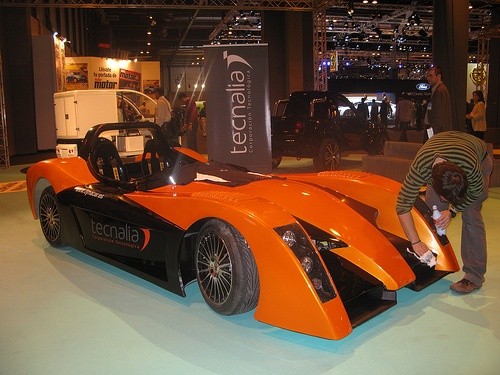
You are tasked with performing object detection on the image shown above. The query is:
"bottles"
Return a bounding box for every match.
[433,205,446,236]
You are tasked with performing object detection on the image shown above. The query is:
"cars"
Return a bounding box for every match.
[343,100,397,124]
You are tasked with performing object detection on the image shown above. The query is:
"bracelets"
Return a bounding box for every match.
[411,239,422,246]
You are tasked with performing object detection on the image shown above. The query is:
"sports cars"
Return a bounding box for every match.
[27,122,461,340]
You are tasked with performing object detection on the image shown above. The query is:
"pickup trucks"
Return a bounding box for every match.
[271,91,389,173]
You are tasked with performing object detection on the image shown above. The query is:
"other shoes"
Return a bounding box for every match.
[450,278,482,294]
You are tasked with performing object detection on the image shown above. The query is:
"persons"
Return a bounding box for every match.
[357,91,390,129]
[416,100,422,131]
[395,130,493,293]
[155,88,173,146]
[397,94,414,139]
[465,91,487,140]
[139,103,150,114]
[178,93,198,152]
[423,67,450,141]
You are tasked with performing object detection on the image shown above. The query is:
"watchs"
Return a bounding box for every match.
[448,209,456,219]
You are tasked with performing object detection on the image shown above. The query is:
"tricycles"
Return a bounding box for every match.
[54,89,157,158]
